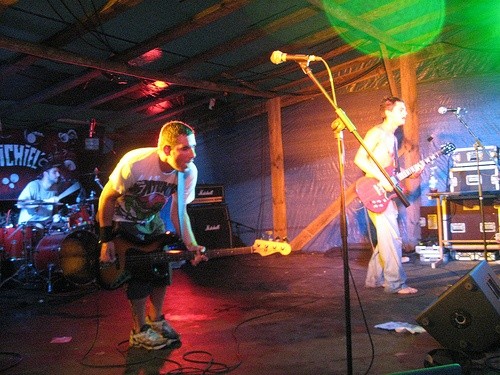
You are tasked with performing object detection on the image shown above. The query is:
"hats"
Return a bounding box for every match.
[42,160,61,172]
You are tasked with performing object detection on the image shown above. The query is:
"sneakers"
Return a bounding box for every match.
[130,325,168,350]
[146,316,180,341]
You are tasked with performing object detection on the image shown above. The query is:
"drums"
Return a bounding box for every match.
[0,204,100,288]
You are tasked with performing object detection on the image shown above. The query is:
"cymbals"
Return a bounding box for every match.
[75,171,109,177]
[77,196,99,201]
[12,200,65,206]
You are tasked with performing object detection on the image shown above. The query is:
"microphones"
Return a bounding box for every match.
[76,189,83,203]
[90,120,94,138]
[437,106,466,114]
[270,51,322,65]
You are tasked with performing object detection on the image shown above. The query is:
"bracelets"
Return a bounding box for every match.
[100,226,114,243]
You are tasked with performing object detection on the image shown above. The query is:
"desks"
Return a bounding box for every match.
[425,192,500,269]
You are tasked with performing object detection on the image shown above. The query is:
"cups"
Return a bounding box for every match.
[429,171,439,193]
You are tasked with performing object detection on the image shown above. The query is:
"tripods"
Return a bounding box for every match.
[0,205,48,290]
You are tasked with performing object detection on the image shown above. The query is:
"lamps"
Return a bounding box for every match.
[84,118,96,138]
[415,260,500,352]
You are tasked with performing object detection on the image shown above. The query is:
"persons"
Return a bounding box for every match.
[17,161,62,225]
[354,96,425,295]
[98,121,208,352]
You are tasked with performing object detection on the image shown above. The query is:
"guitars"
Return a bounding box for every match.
[94,230,293,291]
[354,142,456,213]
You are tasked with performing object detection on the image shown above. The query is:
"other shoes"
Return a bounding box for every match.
[398,287,418,294]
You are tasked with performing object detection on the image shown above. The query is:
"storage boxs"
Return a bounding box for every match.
[449,165,500,192]
[452,145,498,167]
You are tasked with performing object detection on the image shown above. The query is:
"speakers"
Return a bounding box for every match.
[415,260,500,356]
[187,205,232,251]
